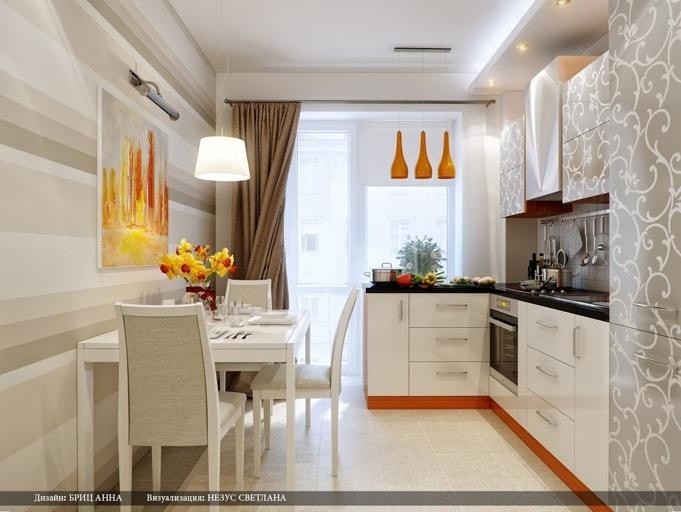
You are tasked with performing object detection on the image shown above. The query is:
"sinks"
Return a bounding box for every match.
[539,292,609,310]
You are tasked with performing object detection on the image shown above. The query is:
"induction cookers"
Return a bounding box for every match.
[505,287,608,296]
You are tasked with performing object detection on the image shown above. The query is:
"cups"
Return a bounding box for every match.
[162,299,175,305]
[205,311,214,323]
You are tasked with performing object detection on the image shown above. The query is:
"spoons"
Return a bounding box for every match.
[232,330,244,339]
[242,330,252,339]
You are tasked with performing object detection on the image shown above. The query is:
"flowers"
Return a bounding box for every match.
[157,238,236,292]
[397,234,446,274]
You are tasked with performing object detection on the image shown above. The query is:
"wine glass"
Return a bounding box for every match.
[191,297,201,304]
[231,295,243,327]
[218,303,227,327]
[216,296,224,310]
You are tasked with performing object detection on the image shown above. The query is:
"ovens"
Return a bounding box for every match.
[489,293,519,396]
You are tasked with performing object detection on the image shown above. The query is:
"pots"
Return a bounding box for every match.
[519,275,557,291]
[364,263,403,284]
[541,263,582,287]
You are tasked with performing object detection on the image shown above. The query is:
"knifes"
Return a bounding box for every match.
[224,331,236,339]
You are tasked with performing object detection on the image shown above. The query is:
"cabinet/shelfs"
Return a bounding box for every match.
[517,291,574,491]
[410,286,490,410]
[363,288,409,409]
[561,49,616,204]
[574,303,608,507]
[499,114,526,218]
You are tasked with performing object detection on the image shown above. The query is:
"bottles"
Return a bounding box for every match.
[528,259,532,279]
[539,253,544,267]
[530,253,537,279]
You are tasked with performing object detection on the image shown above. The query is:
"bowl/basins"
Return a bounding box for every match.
[247,306,262,314]
[257,311,289,319]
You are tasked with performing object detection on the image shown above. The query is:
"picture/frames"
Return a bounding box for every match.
[98,81,172,271]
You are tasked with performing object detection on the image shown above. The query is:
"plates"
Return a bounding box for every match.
[204,323,215,337]
[248,315,298,325]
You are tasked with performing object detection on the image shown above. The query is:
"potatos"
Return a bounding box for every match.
[472,277,495,284]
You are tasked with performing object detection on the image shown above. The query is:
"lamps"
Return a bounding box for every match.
[390,47,456,180]
[194,1,251,182]
